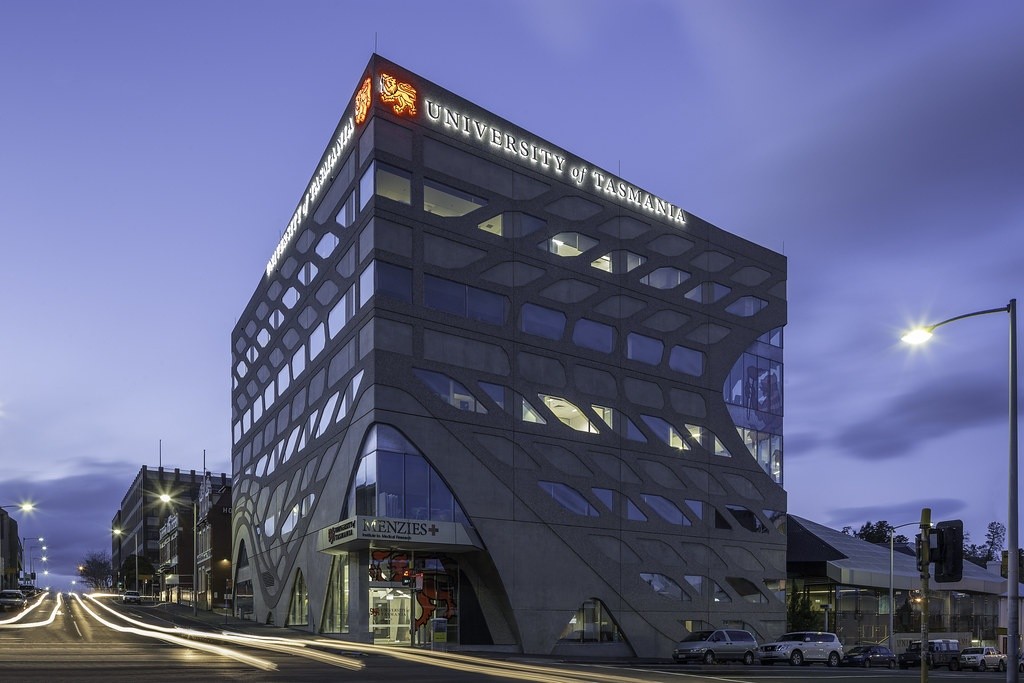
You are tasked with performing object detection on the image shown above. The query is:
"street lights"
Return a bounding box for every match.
[113,530,139,592]
[32,557,47,585]
[900,298,1020,683]
[887,520,935,653]
[23,537,44,588]
[158,493,198,617]
[29,545,46,583]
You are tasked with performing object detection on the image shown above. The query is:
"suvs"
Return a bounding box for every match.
[673,628,760,666]
[897,639,962,671]
[758,631,845,667]
[961,646,1008,672]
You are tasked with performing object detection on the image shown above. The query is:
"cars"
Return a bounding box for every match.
[841,645,897,669]
[0,589,27,611]
[123,591,142,605]
